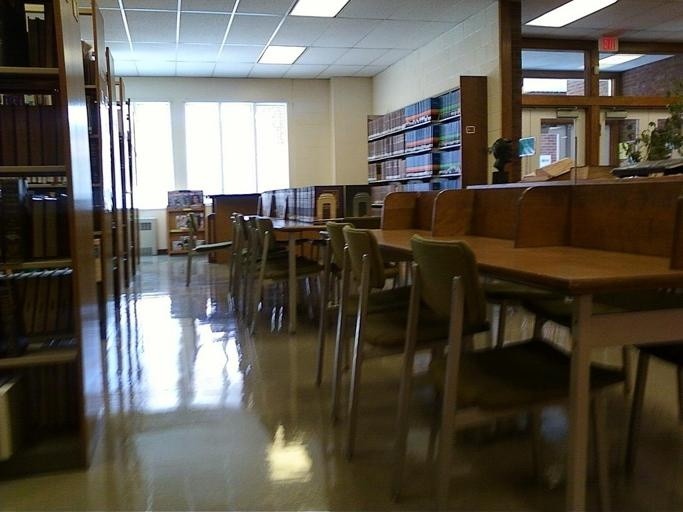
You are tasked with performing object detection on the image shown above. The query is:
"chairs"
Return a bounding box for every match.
[403,239,615,500]
[334,224,425,437]
[214,212,368,392]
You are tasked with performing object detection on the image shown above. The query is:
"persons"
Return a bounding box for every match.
[492,137,513,172]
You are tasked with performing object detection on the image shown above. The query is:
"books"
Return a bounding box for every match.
[367,90,461,204]
[261,185,371,225]
[0,3,102,463]
[522,158,581,182]
[168,191,204,251]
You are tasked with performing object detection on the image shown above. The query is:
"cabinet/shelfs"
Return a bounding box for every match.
[364,75,487,208]
[138,218,158,256]
[1,1,138,471]
[165,204,207,256]
